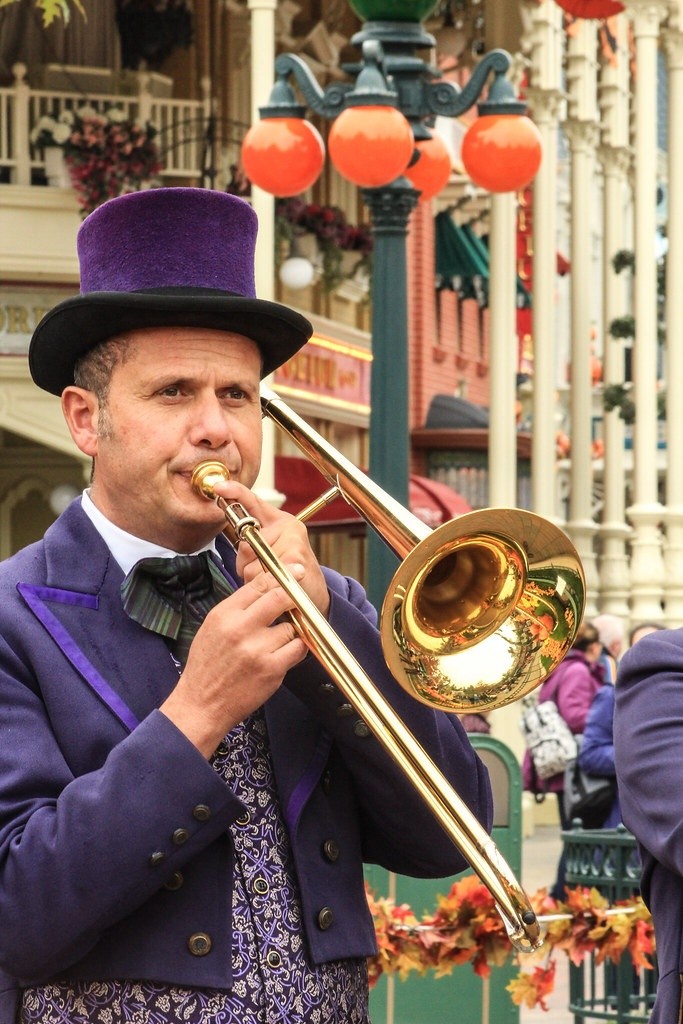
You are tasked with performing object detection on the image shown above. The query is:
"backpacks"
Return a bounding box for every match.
[520,700,578,780]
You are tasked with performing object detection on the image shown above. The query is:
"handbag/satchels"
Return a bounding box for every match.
[558,760,615,830]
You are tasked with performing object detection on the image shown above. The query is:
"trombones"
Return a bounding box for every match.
[193,381,587,954]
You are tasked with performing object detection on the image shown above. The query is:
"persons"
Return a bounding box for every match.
[523,624,607,901]
[612,625,683,1024]
[578,623,673,1014]
[0,186,493,1024]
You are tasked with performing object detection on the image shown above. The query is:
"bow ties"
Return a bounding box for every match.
[118,550,240,640]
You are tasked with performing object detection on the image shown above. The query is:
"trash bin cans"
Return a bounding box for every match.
[357,732,529,1024]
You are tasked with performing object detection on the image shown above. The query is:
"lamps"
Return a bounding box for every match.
[403,123,451,203]
[241,75,325,197]
[461,73,542,192]
[327,66,415,188]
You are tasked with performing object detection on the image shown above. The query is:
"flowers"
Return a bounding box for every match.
[31,107,166,221]
[227,167,371,254]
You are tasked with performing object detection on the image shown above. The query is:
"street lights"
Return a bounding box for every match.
[239,4,543,650]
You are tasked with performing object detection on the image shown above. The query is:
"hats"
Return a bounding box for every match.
[28,187,312,394]
